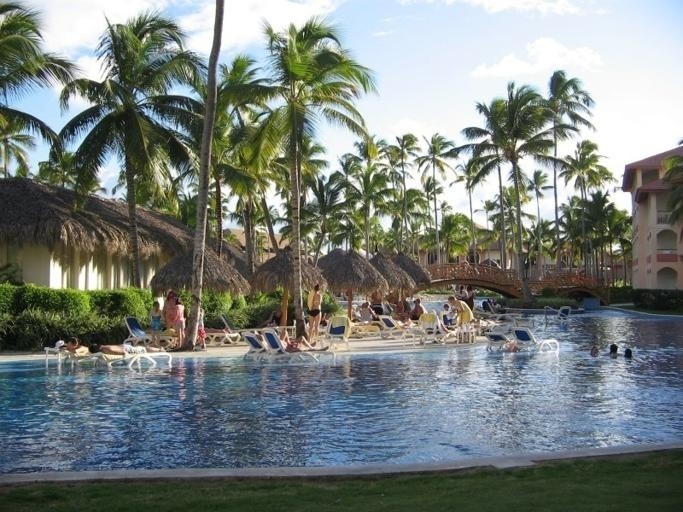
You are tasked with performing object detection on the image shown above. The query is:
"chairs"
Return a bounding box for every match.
[42,301,571,373]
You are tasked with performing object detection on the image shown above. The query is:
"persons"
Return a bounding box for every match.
[55,339,68,350]
[63,336,89,353]
[89,342,167,355]
[256,327,330,353]
[359,284,475,335]
[306,283,323,341]
[320,312,361,326]
[591,344,634,359]
[148,290,207,351]
